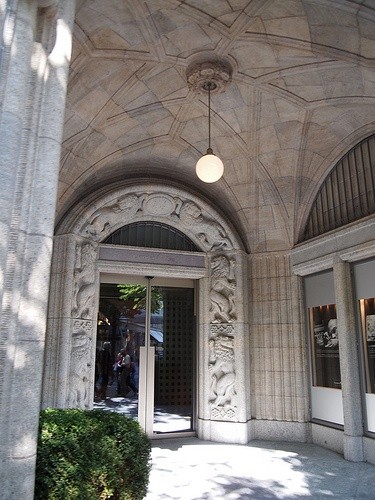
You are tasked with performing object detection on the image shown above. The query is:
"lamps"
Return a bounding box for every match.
[187,60,233,184]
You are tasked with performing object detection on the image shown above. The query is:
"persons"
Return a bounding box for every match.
[314,317,374,349]
[93,339,139,404]
